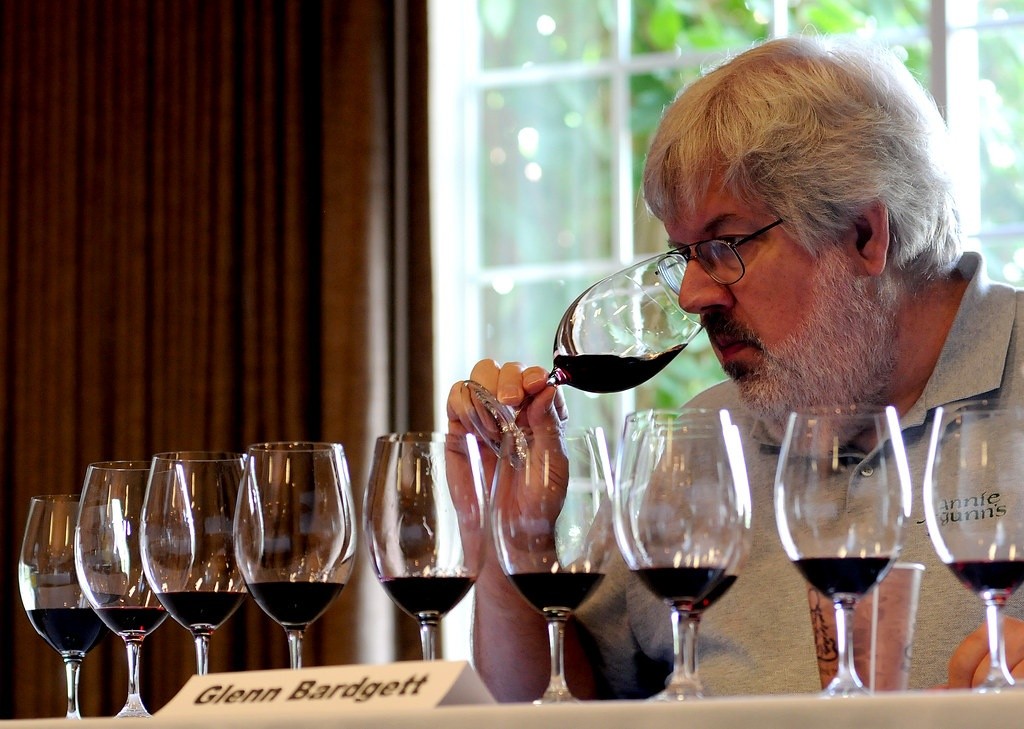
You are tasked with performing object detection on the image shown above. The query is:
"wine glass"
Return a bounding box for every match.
[461,251,706,470]
[773,407,909,699]
[139,452,264,674]
[619,407,742,699]
[361,433,490,663]
[921,398,1024,692]
[230,443,358,670]
[17,494,112,720]
[73,461,196,718]
[634,422,753,699]
[489,423,619,706]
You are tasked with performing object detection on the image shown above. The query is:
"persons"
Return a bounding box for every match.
[444,24,1024,704]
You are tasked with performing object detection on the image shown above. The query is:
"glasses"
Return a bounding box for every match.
[656,216,785,297]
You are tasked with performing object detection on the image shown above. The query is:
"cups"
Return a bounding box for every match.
[804,561,925,696]
